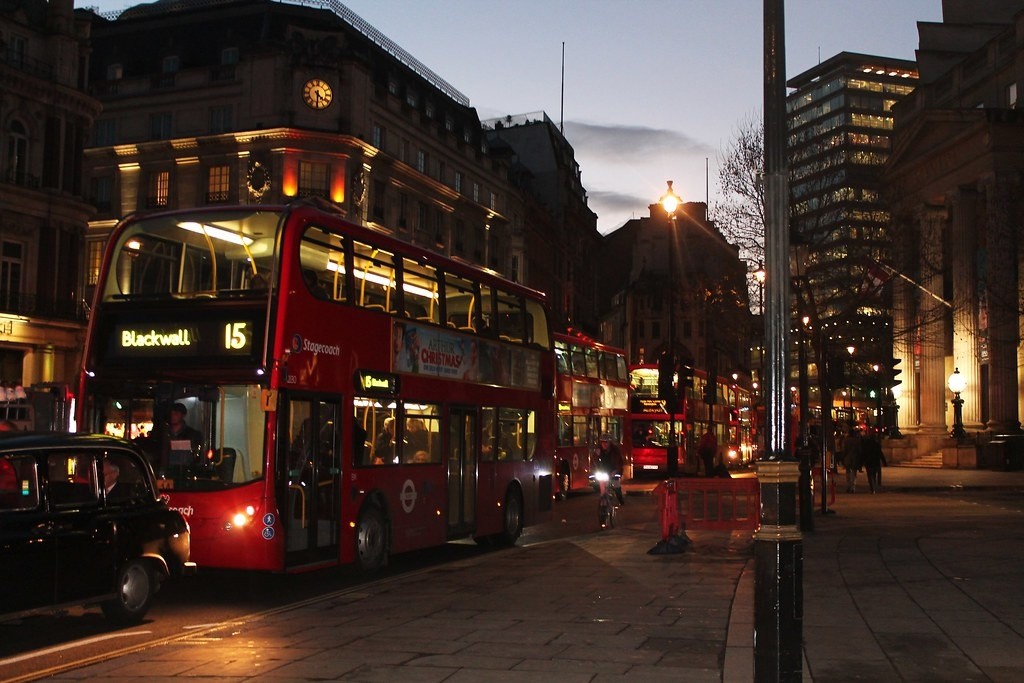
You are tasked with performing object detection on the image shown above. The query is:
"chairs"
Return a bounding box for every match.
[174,291,518,342]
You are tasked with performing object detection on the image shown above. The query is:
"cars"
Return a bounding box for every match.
[0,430,197,629]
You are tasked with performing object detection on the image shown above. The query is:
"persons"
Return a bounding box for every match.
[165,403,203,490]
[482,422,514,460]
[374,417,431,465]
[697,425,718,477]
[596,433,626,517]
[824,425,888,495]
[80,458,126,512]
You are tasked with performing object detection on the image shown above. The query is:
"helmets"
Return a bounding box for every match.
[599,433,613,441]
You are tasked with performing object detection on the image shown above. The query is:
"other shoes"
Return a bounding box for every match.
[620,496,625,504]
[871,490,876,494]
[852,488,855,493]
[601,511,606,521]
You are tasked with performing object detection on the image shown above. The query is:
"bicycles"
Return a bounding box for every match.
[588,473,622,531]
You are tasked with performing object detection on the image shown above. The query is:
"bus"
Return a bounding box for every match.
[72,199,558,585]
[629,364,758,478]
[790,402,838,462]
[551,331,634,503]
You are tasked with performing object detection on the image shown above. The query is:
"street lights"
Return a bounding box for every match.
[658,179,684,479]
[845,346,856,422]
[948,367,968,439]
[751,260,765,398]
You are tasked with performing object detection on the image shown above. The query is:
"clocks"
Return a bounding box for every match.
[303,78,333,109]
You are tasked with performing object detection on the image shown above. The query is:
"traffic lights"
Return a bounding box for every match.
[881,356,903,389]
[676,357,695,389]
